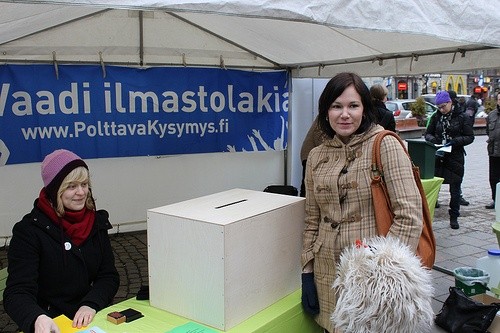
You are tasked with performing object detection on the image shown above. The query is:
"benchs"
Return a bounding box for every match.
[395,117,487,140]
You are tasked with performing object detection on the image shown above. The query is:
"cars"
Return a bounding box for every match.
[384,98,439,119]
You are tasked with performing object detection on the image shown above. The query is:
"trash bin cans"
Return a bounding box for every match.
[454,267,489,297]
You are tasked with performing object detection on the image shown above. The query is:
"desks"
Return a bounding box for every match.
[88,288,323,333]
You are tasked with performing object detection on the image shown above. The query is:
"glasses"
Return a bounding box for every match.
[438,103,448,110]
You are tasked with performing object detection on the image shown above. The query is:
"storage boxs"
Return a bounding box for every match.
[146,188,307,332]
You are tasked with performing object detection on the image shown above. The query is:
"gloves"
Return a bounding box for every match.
[302,271,320,315]
[444,141,454,154]
[431,138,442,144]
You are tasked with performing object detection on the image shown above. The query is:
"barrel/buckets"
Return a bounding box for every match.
[476,249,500,290]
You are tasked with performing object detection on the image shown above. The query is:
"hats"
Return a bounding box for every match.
[435,91,451,105]
[41,149,89,208]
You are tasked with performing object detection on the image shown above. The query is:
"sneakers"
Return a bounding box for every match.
[435,200,439,208]
[459,197,469,205]
[486,201,495,209]
[450,218,459,229]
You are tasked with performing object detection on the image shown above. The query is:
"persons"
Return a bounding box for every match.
[301,71,423,333]
[423,91,474,229]
[435,89,480,207]
[300,114,333,198]
[485,92,500,209]
[370,83,397,133]
[2,148,120,333]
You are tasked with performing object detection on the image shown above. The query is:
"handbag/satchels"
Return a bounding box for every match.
[465,107,474,116]
[435,151,449,163]
[371,130,436,270]
[434,286,500,333]
